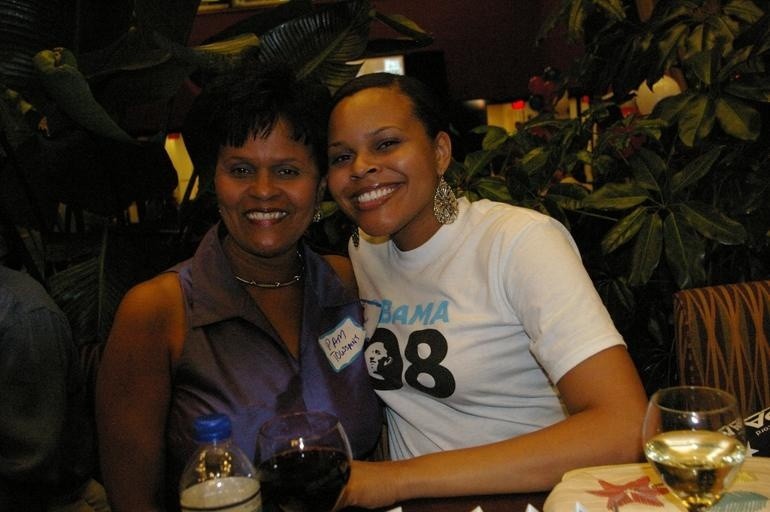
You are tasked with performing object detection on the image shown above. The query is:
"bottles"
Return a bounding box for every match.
[178,411,262,512]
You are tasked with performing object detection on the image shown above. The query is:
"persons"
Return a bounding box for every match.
[92,70,383,510]
[309,76,661,511]
[0,265,107,509]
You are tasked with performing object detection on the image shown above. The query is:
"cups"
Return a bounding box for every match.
[253,412,353,512]
[641,387,741,510]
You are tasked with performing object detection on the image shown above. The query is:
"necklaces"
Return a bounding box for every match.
[220,235,307,289]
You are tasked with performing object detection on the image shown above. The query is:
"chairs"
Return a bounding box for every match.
[670,277,770,436]
